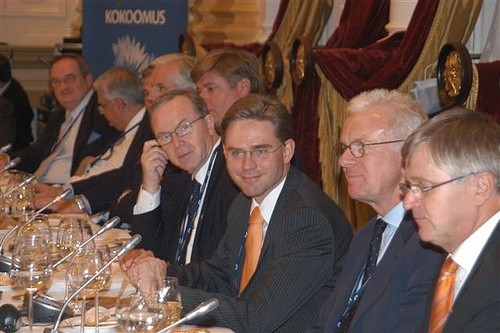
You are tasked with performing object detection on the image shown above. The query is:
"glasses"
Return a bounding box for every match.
[97,99,127,111]
[333,140,407,158]
[226,142,285,159]
[157,115,205,146]
[50,72,84,87]
[398,171,490,201]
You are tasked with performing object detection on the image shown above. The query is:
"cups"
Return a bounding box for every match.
[114,277,181,333]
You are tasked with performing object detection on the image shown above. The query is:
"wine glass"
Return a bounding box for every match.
[0,171,112,333]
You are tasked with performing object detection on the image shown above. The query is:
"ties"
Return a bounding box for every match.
[239,206,265,294]
[341,219,389,332]
[36,115,73,180]
[178,177,202,264]
[427,257,458,333]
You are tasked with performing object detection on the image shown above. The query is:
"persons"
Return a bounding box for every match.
[0,51,500,333]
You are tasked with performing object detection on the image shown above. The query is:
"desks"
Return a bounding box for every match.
[0,214,236,333]
